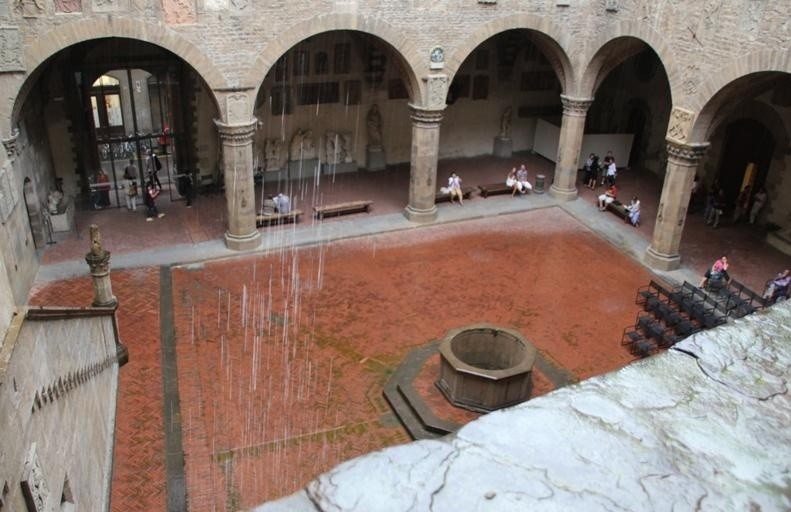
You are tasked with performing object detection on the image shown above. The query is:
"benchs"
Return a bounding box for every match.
[598,201,631,223]
[478,183,525,199]
[435,187,471,204]
[256,209,304,224]
[313,200,374,219]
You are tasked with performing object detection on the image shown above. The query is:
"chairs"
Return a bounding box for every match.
[621,268,786,358]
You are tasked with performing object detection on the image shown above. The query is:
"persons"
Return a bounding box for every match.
[697,254,729,289]
[622,194,640,227]
[516,164,532,195]
[251,99,386,172]
[122,158,139,198]
[274,193,290,214]
[597,181,617,211]
[506,166,522,200]
[94,169,112,207]
[124,174,137,212]
[584,149,617,190]
[159,128,171,153]
[762,267,791,303]
[448,171,464,207]
[144,180,166,222]
[499,106,514,140]
[257,194,276,217]
[680,169,768,231]
[144,149,163,190]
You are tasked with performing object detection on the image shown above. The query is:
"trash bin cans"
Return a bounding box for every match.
[534,174,545,194]
